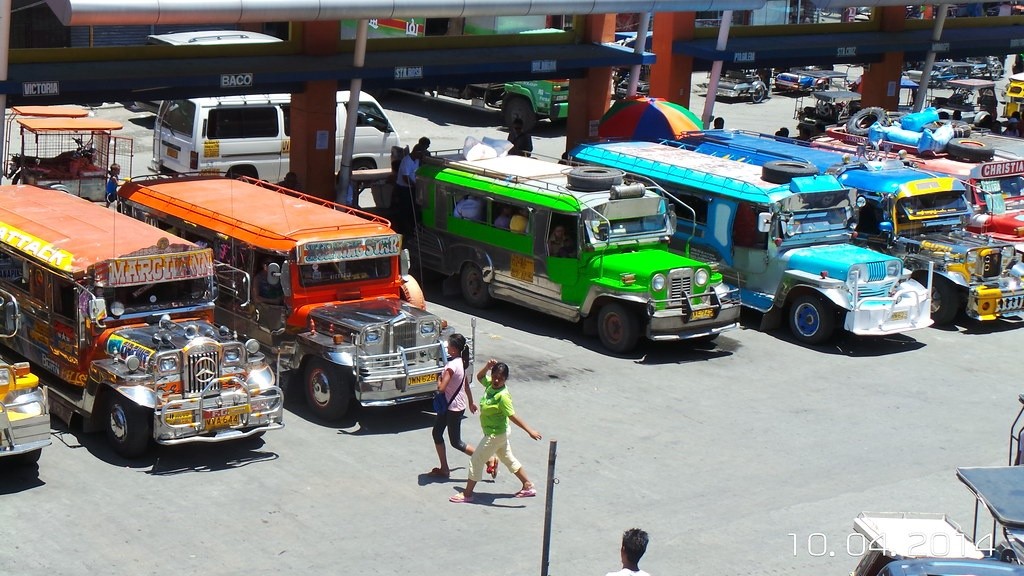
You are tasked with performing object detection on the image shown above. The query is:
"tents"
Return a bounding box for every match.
[900,77,920,106]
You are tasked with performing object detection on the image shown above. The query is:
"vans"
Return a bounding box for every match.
[148,96,402,184]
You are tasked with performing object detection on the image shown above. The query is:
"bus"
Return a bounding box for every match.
[658,129,1024,324]
[119,162,478,426]
[565,136,935,347]
[809,119,1024,259]
[0,182,285,464]
[408,147,742,353]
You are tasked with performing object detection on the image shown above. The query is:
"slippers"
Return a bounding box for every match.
[513,489,537,498]
[449,493,474,502]
[429,467,449,477]
[486,457,498,479]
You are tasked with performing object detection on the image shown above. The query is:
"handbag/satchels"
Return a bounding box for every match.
[432,393,447,416]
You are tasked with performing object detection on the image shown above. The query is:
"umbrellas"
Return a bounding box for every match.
[597,96,703,142]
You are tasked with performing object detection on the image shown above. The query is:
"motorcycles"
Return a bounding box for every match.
[8,105,133,202]
[792,56,1024,137]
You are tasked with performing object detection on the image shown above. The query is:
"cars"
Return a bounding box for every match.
[706,63,773,103]
[776,65,834,95]
[615,72,649,98]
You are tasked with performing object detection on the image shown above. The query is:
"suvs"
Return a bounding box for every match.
[146,31,283,46]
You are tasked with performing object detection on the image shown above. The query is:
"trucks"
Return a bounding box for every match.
[338,12,569,132]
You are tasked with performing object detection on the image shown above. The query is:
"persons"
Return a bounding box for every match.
[775,122,825,141]
[429,333,498,478]
[713,117,724,129]
[395,145,422,232]
[449,359,542,502]
[104,163,124,208]
[938,110,1024,137]
[507,118,533,158]
[604,528,652,576]
[418,137,431,163]
